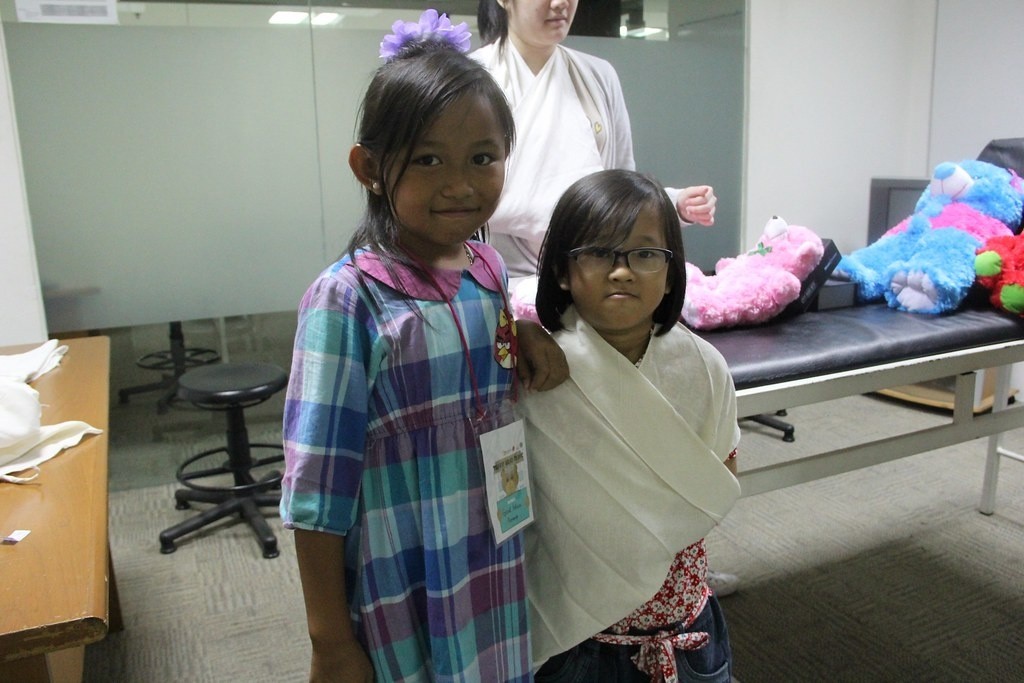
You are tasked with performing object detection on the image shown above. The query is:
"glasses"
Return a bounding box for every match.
[569,246,673,274]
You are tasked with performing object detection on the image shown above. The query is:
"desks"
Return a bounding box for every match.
[1,335,124,683]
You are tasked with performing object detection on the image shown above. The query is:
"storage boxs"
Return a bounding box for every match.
[817,281,857,311]
[785,239,842,314]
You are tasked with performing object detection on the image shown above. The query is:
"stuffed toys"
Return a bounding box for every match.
[508,261,541,326]
[975,229,1024,318]
[681,217,825,329]
[831,159,1024,316]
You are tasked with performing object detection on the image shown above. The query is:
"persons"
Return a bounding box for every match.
[514,169,743,683]
[278,7,571,683]
[465,0,718,278]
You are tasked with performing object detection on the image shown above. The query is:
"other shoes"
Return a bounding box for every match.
[708,570,740,597]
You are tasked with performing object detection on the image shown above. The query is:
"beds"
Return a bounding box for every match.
[679,298,1024,515]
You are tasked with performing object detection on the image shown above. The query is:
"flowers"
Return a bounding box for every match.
[379,9,472,60]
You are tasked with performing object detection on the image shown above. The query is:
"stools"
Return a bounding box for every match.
[160,363,287,558]
